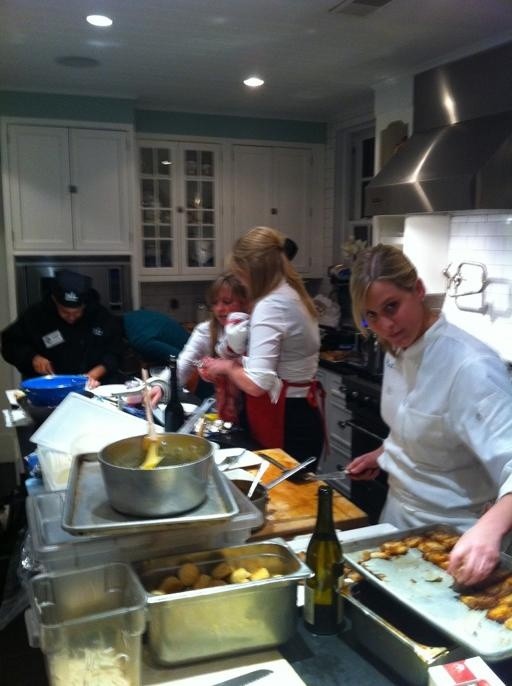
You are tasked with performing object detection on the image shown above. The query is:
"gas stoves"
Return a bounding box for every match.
[335,366,387,422]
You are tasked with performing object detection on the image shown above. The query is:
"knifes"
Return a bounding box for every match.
[306,469,352,482]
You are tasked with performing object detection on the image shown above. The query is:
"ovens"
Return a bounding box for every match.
[339,405,388,525]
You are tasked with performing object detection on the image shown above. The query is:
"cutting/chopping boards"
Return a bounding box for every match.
[219,447,369,541]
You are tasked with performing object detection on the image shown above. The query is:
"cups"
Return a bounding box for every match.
[194,240,210,266]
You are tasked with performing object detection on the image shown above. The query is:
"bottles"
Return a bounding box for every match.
[300,487,346,635]
[165,354,185,431]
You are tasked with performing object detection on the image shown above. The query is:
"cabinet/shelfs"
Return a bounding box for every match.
[5,120,139,259]
[218,138,318,276]
[318,369,353,497]
[132,131,222,281]
[370,215,452,295]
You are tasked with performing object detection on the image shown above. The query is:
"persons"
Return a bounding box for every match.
[143,271,255,444]
[1,267,126,389]
[116,309,189,371]
[342,238,512,589]
[193,226,325,474]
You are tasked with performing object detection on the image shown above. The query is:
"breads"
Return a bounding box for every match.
[151,562,282,597]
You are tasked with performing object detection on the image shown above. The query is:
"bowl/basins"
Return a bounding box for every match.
[20,371,91,405]
[179,402,198,415]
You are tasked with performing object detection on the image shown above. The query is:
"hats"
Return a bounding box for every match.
[50,270,87,308]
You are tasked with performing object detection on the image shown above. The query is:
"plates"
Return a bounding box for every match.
[215,440,263,476]
[94,381,146,405]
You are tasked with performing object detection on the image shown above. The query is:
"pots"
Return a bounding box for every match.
[227,453,317,533]
[91,394,220,519]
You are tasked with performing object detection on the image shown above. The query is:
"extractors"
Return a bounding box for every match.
[363,43,511,220]
